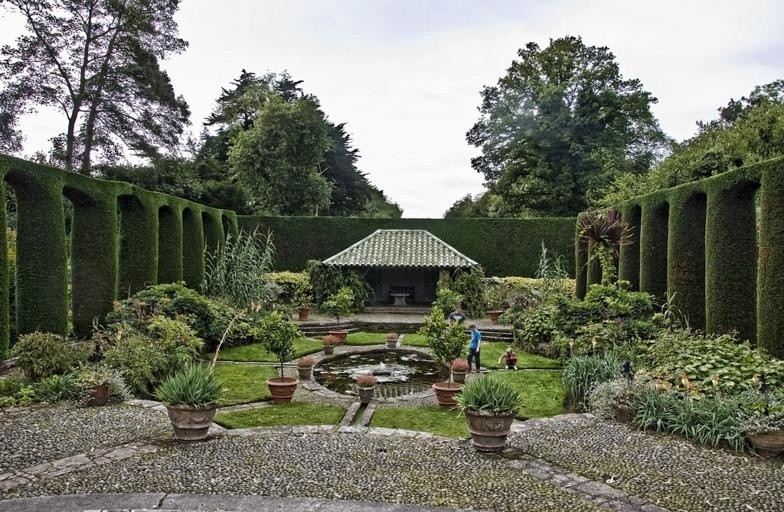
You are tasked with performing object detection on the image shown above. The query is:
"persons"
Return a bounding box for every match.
[465,324,482,373]
[497,347,518,370]
[447,305,466,327]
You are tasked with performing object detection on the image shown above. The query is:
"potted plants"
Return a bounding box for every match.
[451,358,468,382]
[418,304,470,409]
[385,334,398,348]
[482,286,503,322]
[588,379,642,423]
[296,356,314,379]
[155,362,230,442]
[729,407,783,456]
[322,336,338,355]
[68,362,133,408]
[253,303,304,403]
[356,375,377,402]
[455,374,522,453]
[292,284,312,320]
[323,286,353,342]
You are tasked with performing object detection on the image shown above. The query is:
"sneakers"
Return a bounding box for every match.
[505,365,517,370]
[476,368,480,372]
